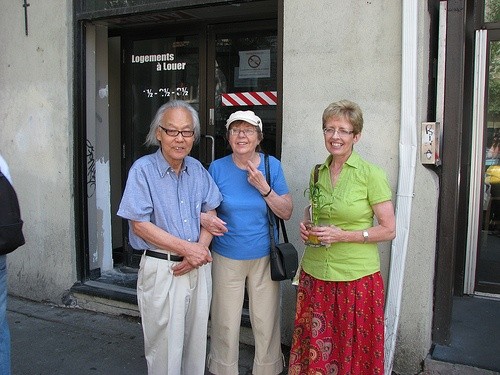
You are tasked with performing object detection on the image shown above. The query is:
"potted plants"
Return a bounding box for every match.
[304,181,334,248]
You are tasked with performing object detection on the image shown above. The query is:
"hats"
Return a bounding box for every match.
[225,111,262,132]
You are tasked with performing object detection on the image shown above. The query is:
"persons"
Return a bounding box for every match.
[200,110,294,375]
[0,155,12,375]
[287,99,396,375]
[117,100,223,375]
[481,141,500,211]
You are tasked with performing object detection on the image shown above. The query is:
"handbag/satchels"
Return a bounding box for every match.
[270,242,299,282]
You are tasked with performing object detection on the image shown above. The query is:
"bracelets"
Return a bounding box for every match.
[261,187,271,197]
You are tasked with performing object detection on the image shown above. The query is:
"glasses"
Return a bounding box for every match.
[229,128,257,135]
[158,125,194,137]
[323,128,354,137]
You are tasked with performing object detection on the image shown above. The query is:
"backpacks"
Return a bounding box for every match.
[0,171,26,257]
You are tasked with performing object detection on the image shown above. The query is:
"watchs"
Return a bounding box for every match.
[360,230,368,244]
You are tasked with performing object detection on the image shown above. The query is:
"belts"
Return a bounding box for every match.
[143,250,184,261]
